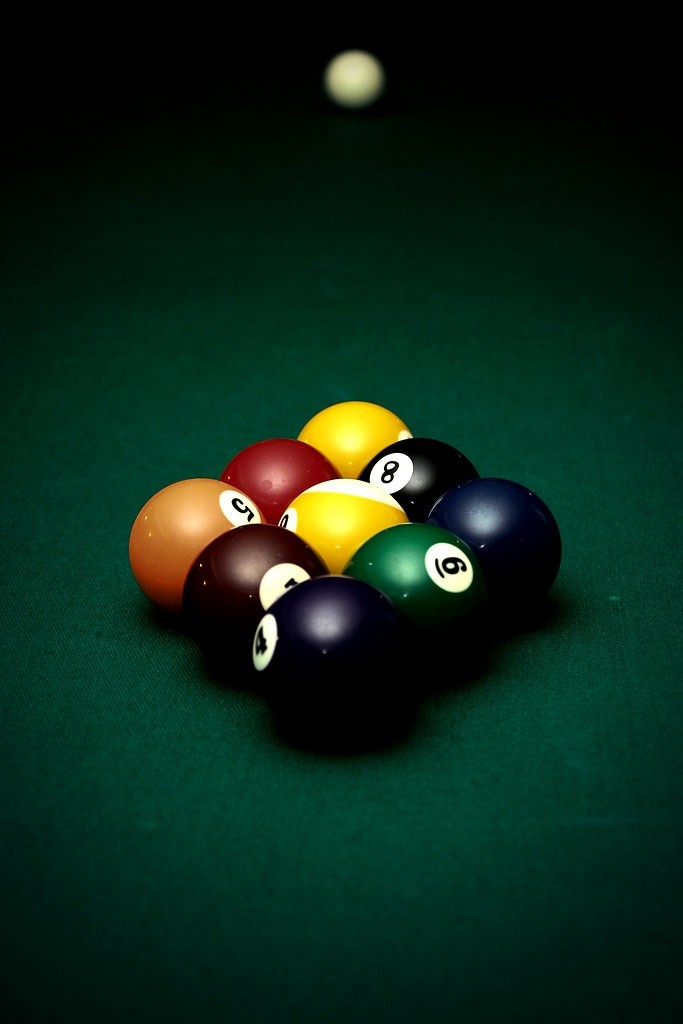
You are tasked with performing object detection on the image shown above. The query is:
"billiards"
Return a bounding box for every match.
[128,399,565,732]
[327,51,382,103]
